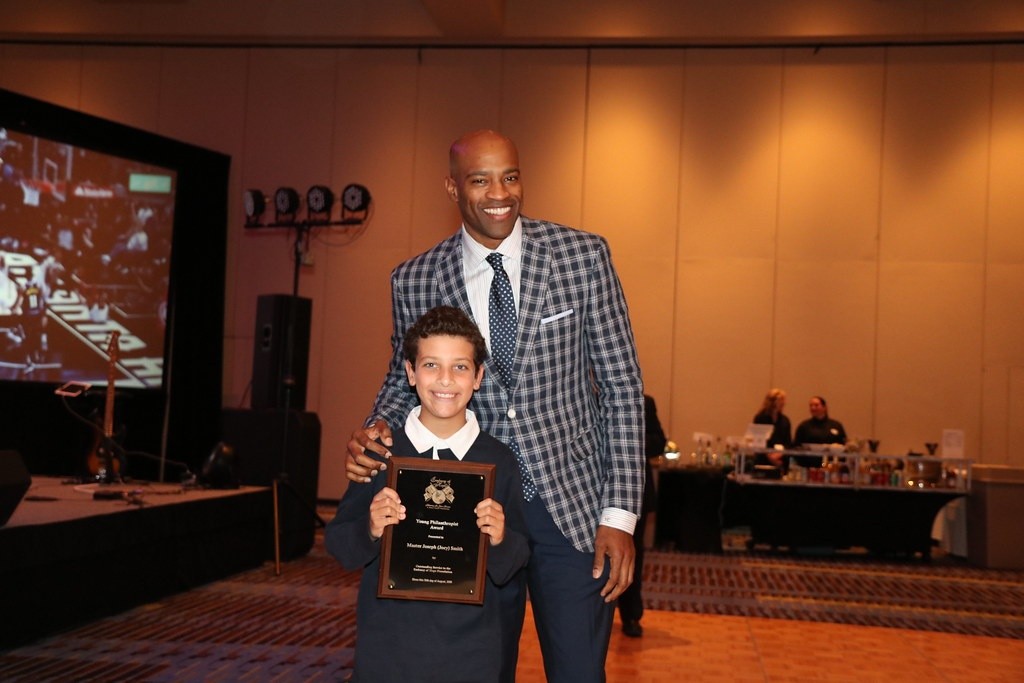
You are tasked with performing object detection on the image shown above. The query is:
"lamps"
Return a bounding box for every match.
[242,189,266,224]
[306,185,334,220]
[274,188,300,222]
[341,183,370,219]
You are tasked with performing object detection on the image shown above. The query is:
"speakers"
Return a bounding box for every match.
[225,406,322,562]
[251,294,312,410]
[0,449,31,529]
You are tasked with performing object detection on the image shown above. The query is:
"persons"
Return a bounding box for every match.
[748,388,792,477]
[324,308,532,683]
[344,131,646,683]
[0,155,154,322]
[792,396,847,467]
[616,396,667,636]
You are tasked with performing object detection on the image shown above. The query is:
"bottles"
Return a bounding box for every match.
[787,439,971,490]
[693,435,754,476]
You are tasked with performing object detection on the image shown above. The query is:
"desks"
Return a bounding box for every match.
[658,466,784,558]
[720,474,976,566]
